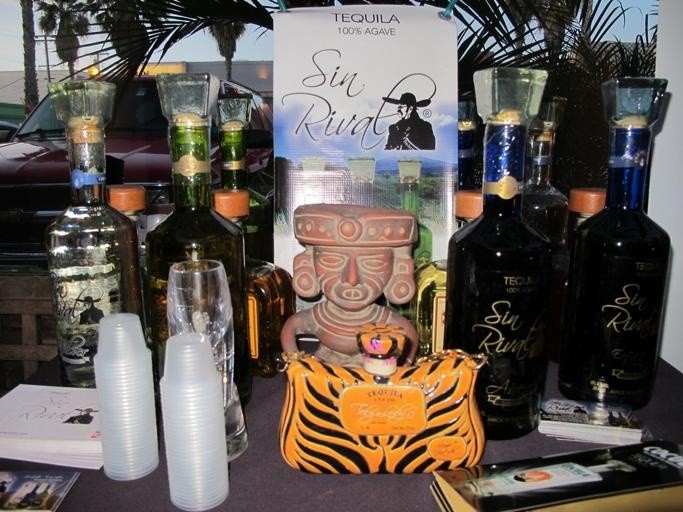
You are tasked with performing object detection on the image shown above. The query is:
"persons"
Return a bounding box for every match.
[280,202,421,365]
[76,295,105,326]
[379,93,439,153]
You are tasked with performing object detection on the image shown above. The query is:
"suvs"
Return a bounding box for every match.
[1,76,273,274]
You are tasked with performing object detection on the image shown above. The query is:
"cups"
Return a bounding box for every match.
[157,331,230,512]
[89,310,160,483]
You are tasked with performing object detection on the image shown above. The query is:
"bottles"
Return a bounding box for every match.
[212,191,278,378]
[411,190,483,356]
[102,182,147,270]
[523,95,571,355]
[557,77,672,409]
[443,66,554,441]
[566,187,606,232]
[208,88,271,269]
[148,67,257,409]
[33,76,148,390]
[297,157,432,319]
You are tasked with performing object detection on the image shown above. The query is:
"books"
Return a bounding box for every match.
[0,382,118,511]
[428,395,683,511]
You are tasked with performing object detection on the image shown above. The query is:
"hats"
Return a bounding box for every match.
[381,93,431,107]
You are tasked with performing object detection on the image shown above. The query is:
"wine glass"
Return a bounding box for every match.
[165,258,231,353]
